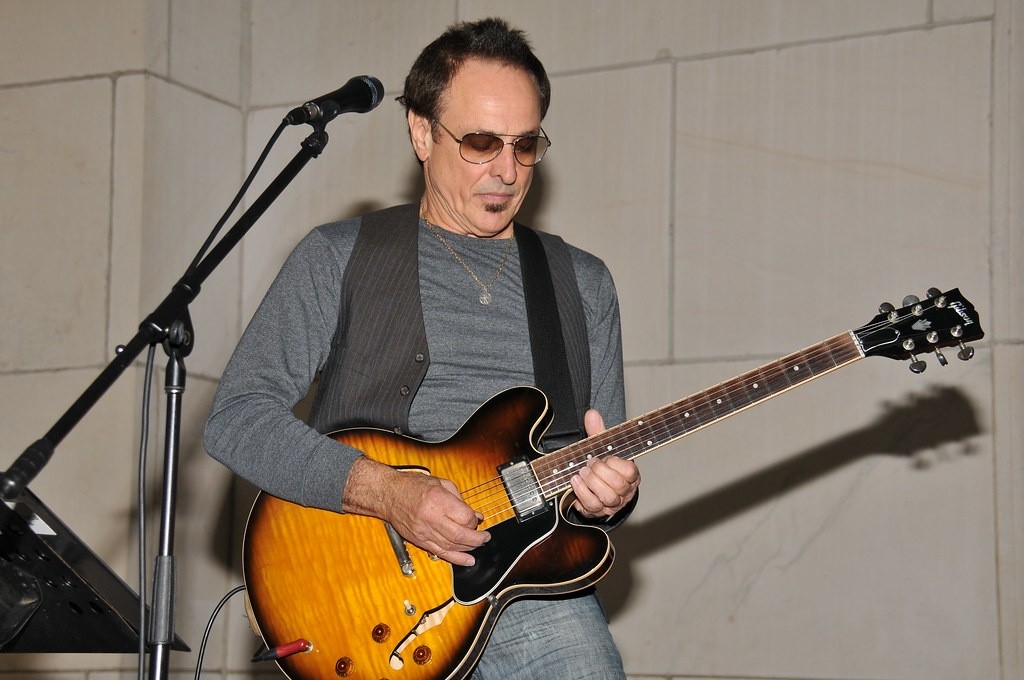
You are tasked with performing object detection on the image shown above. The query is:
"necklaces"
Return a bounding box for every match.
[421,196,513,305]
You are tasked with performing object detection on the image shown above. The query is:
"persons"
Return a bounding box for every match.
[204,15,640,680]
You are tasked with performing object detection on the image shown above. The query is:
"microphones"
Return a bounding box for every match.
[284,75,384,125]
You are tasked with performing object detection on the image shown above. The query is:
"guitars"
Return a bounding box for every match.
[239,288,985,680]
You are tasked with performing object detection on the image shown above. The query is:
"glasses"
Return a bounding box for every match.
[395,94,552,167]
